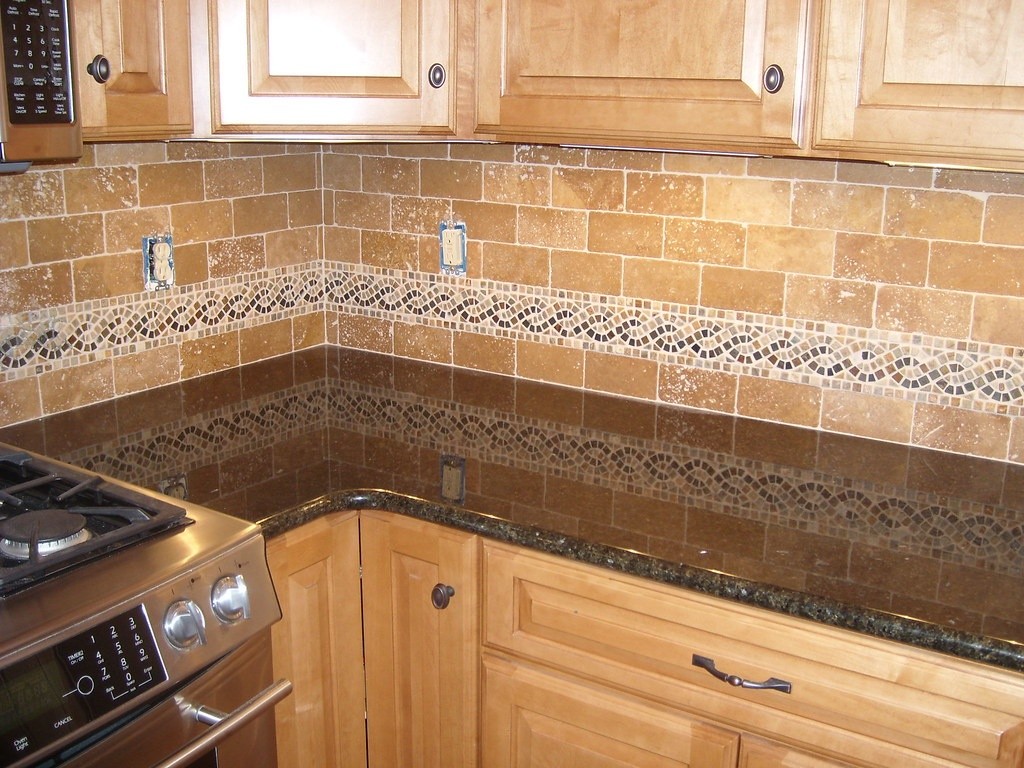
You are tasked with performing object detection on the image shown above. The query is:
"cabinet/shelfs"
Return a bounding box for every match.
[362,508,481,765]
[68,0,194,140]
[265,510,366,767]
[197,0,477,143]
[481,535,1022,767]
[478,0,1024,168]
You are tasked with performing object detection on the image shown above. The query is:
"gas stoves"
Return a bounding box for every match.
[0,442,284,767]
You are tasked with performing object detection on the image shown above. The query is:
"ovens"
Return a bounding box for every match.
[46,624,292,768]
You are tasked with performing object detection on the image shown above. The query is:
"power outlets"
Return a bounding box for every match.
[439,227,465,270]
[143,235,175,290]
[439,457,464,505]
